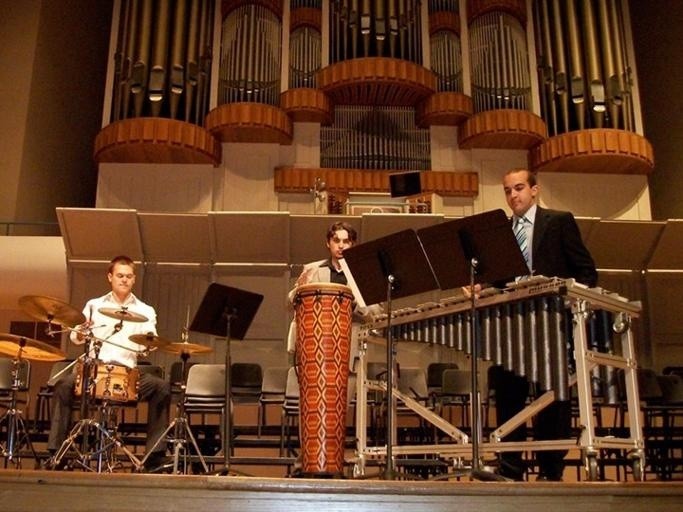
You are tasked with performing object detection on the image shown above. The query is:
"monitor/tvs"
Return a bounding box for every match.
[388,170,421,198]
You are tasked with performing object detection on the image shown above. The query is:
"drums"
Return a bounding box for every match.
[74,359,139,402]
[292,282,356,477]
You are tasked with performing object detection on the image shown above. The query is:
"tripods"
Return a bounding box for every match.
[349,282,424,481]
[429,267,516,482]
[201,314,253,477]
[45,341,144,474]
[132,353,209,475]
[0,356,39,469]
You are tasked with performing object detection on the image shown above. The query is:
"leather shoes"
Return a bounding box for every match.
[146,452,181,472]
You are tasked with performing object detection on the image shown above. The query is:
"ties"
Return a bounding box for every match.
[514,218,528,262]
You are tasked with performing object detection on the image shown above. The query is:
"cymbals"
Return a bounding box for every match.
[159,342,211,354]
[19,293,86,325]
[0,333,66,361]
[98,307,149,322]
[128,334,170,347]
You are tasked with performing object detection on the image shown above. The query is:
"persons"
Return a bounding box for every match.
[461,166,602,486]
[288,220,384,479]
[42,255,175,473]
